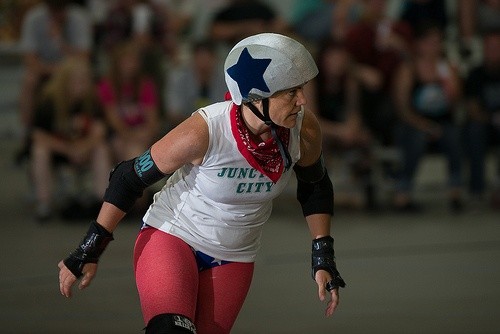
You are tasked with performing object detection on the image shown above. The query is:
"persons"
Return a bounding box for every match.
[19,2,500,220]
[58,32,347,334]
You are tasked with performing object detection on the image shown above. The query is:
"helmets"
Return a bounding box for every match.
[224,32,320,105]
